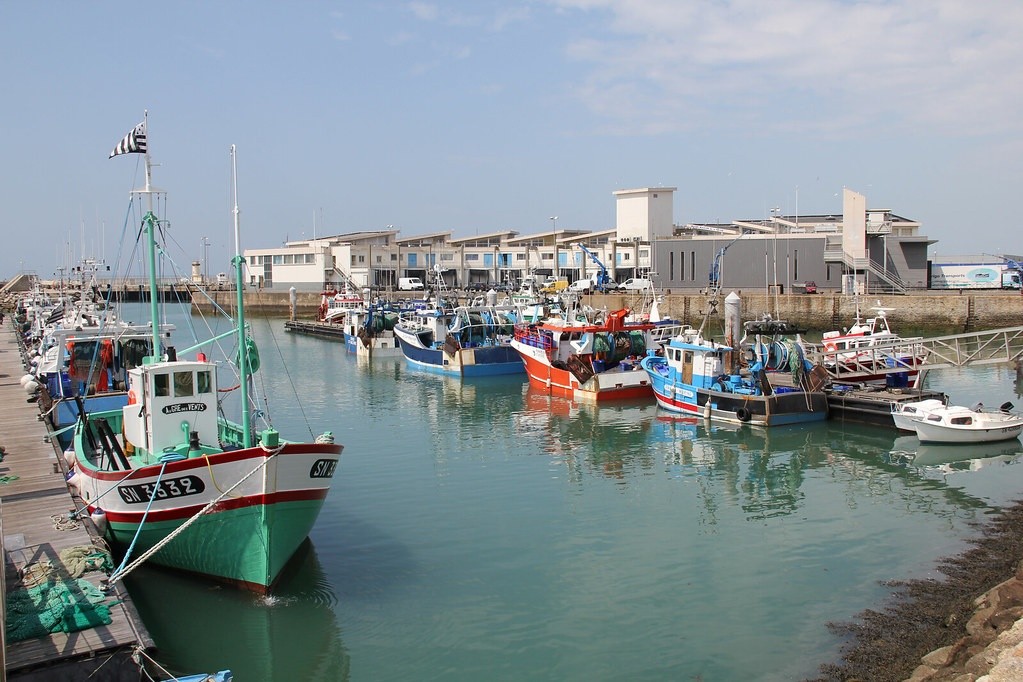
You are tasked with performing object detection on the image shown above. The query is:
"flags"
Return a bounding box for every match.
[109,122,146,159]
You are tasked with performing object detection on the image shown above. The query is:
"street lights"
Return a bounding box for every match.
[770,206,780,285]
[550,216,560,276]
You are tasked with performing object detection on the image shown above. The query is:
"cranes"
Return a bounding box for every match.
[577,242,609,293]
[709,229,751,291]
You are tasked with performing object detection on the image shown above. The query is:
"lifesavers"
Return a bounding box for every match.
[127,391,136,406]
[737,408,747,420]
[824,343,838,357]
[740,408,750,421]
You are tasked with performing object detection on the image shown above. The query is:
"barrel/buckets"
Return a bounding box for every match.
[461,341,476,348]
[886,358,908,387]
[619,361,631,371]
[592,361,606,373]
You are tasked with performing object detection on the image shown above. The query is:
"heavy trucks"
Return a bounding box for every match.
[931,263,1023,290]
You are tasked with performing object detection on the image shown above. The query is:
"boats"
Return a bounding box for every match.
[315,266,683,400]
[890,399,946,432]
[640,228,828,426]
[803,252,924,388]
[911,395,1023,443]
[5,110,344,595]
[113,540,350,682]
[888,434,1023,483]
[743,253,808,335]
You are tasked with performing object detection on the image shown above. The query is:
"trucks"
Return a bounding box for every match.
[794,280,818,294]
[618,278,648,290]
[522,275,594,295]
[398,277,424,291]
[591,276,619,291]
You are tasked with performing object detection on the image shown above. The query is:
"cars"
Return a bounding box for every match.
[463,281,501,292]
